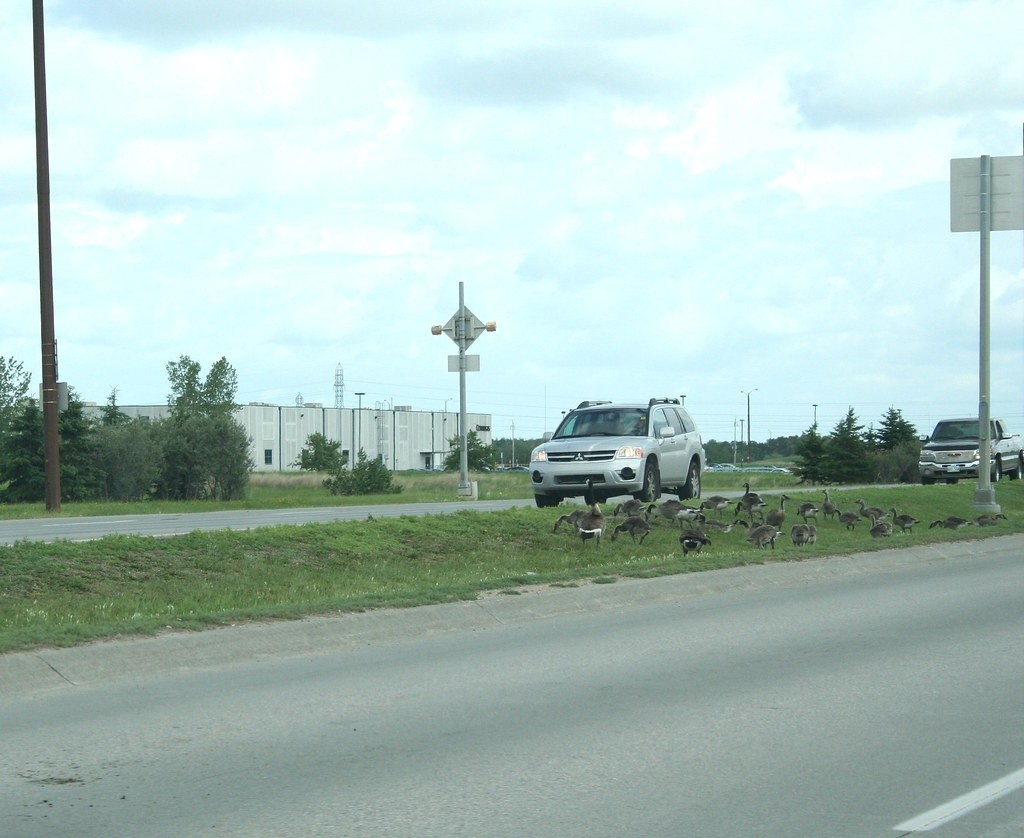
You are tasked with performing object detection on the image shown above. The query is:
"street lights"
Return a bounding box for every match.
[355,393,365,453]
[812,404,818,424]
[384,400,391,410]
[740,419,745,462]
[740,388,758,463]
[445,398,453,413]
[680,395,686,406]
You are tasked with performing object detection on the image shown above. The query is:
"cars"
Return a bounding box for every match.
[705,463,793,473]
[412,465,529,472]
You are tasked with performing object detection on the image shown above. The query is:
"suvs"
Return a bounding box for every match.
[529,397,706,509]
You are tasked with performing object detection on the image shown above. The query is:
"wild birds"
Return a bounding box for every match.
[554,480,1004,554]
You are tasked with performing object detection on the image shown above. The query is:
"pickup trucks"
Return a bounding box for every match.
[919,418,1024,485]
